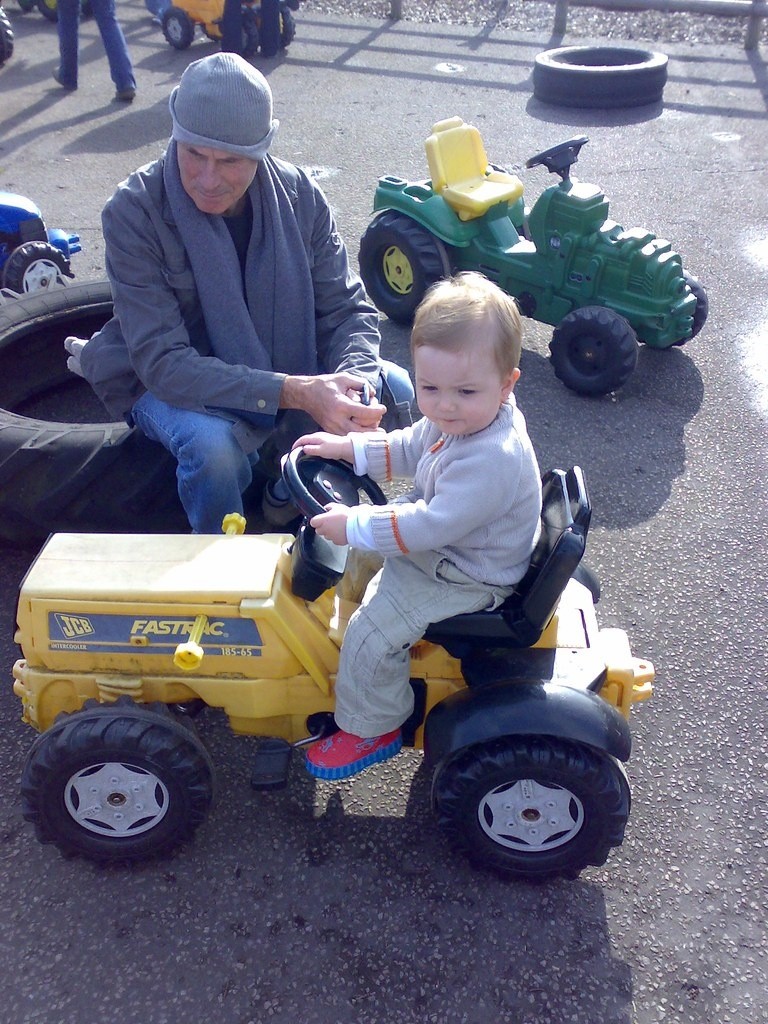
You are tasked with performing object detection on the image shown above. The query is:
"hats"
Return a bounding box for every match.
[170,52,279,162]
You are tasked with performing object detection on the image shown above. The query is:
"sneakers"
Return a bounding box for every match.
[304,729,402,779]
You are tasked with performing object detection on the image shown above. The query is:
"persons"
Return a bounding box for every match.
[291,271,544,780]
[52,1,415,535]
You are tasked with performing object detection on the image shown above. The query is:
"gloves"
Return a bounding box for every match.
[64,331,102,377]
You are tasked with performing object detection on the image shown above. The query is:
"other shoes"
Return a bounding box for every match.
[256,47,276,56]
[116,89,135,100]
[152,18,163,27]
[52,65,77,90]
